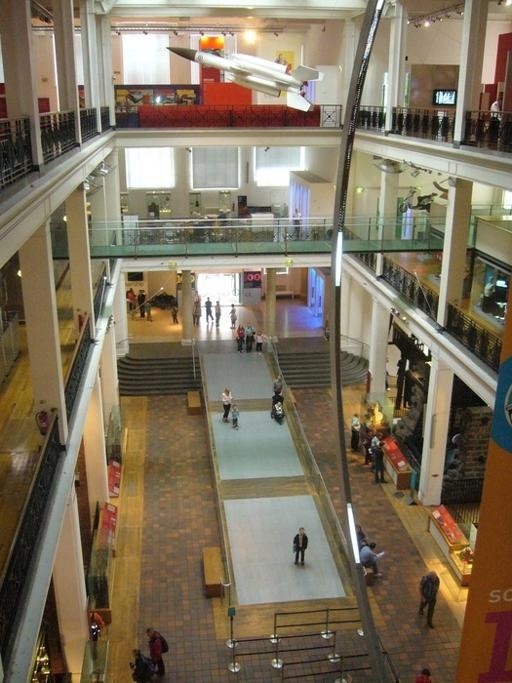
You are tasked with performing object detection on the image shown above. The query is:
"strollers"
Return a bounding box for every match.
[271,394,285,425]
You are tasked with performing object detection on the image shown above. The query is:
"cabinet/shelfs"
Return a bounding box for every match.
[378,430,413,490]
[422,500,473,588]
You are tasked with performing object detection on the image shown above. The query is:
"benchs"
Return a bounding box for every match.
[201,547,224,599]
[186,390,203,417]
[361,561,376,588]
[289,389,297,407]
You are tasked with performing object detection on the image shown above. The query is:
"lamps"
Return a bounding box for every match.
[406,1,464,31]
[107,21,287,37]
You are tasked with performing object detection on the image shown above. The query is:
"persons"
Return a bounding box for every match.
[355,524,385,577]
[419,571,439,629]
[88,613,107,656]
[416,669,432,682]
[293,208,301,237]
[272,392,284,420]
[130,628,165,683]
[222,387,231,423]
[490,98,502,148]
[273,376,284,393]
[126,288,268,355]
[218,210,228,227]
[232,404,239,430]
[352,413,385,483]
[293,528,308,565]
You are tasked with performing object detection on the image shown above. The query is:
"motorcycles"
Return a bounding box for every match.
[399,186,438,213]
